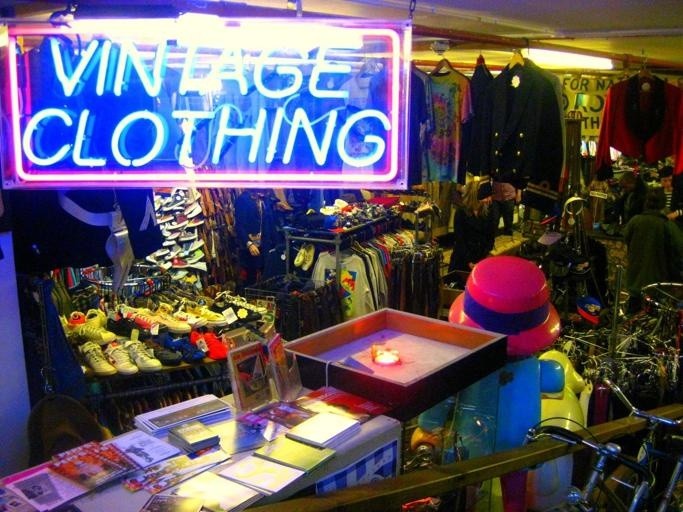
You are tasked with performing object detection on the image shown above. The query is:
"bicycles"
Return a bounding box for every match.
[522,375,683,512]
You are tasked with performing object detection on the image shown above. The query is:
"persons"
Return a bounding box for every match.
[617,166,683,313]
[491,178,521,234]
[234,189,274,284]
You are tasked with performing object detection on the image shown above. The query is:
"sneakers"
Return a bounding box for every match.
[63,290,268,377]
[293,242,316,273]
[145,186,209,271]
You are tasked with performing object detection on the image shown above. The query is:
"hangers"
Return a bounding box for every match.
[608,56,683,95]
[320,211,413,261]
[427,35,551,86]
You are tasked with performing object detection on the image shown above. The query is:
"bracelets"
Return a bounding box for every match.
[246,240,254,248]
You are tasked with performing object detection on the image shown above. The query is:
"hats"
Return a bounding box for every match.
[576,296,601,325]
